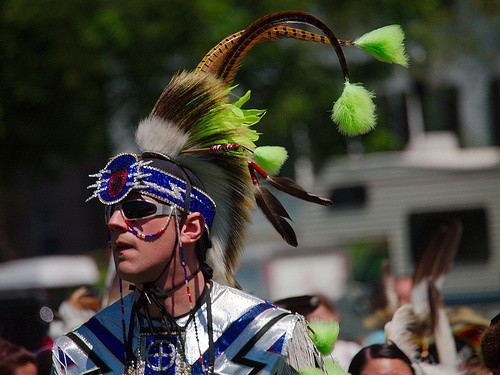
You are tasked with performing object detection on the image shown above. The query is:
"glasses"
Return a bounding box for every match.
[104,198,186,225]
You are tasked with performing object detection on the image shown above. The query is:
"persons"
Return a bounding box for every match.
[1,295,499,375]
[50,154,329,375]
